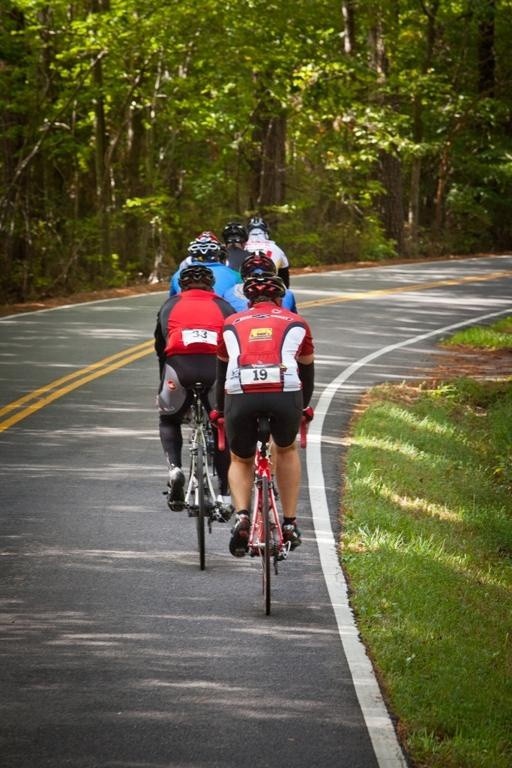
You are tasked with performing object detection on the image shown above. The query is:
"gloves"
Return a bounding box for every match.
[209,409,225,429]
[300,405,314,424]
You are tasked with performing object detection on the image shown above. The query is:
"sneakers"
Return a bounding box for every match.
[166,466,186,512]
[282,519,300,552]
[229,513,251,558]
[212,504,235,523]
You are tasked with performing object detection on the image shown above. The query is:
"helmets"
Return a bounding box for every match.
[193,230,220,244]
[239,250,278,282]
[243,272,287,301]
[220,222,249,243]
[248,216,267,233]
[177,265,216,288]
[187,238,221,259]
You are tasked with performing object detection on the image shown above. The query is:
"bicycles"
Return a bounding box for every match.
[165,380,217,573]
[210,404,315,618]
